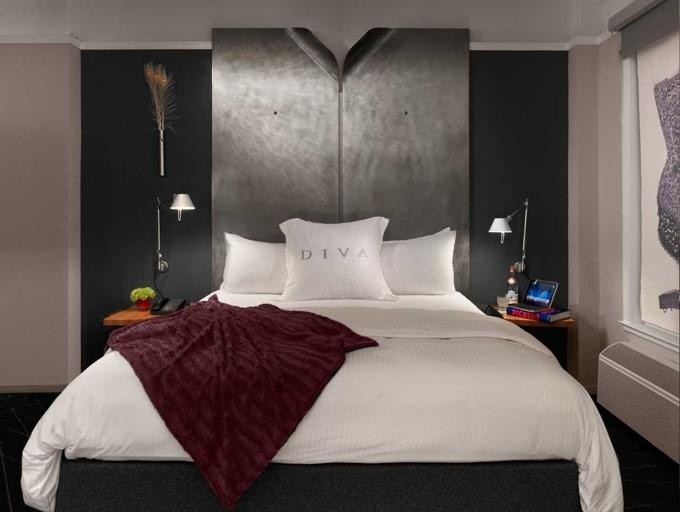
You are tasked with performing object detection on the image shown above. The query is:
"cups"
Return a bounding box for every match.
[497,294,509,311]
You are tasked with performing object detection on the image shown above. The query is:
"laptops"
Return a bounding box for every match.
[507,276,559,313]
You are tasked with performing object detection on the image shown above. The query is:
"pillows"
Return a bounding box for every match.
[219,232,287,294]
[278,216,396,303]
[378,228,459,296]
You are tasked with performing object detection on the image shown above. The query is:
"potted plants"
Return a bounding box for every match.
[129,287,157,312]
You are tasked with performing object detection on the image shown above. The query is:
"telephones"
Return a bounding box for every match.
[151,296,186,316]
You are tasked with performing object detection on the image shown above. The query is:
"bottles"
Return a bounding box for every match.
[505,267,519,304]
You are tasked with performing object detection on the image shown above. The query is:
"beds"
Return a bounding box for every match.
[19,290,625,512]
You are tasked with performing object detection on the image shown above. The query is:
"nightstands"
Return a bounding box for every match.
[101,305,164,326]
[487,304,574,372]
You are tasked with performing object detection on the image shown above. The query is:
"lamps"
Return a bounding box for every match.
[489,194,529,269]
[154,192,194,273]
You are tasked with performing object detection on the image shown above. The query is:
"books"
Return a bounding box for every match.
[507,304,572,324]
